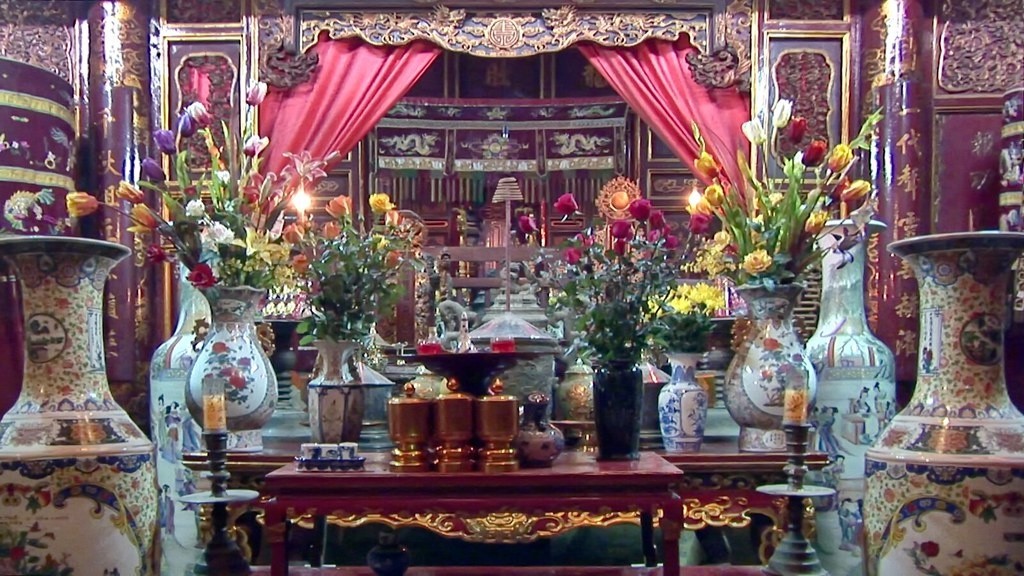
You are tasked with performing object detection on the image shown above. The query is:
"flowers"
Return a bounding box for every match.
[63,77,416,336]
[519,94,888,357]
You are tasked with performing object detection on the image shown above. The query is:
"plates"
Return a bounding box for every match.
[297,457,366,471]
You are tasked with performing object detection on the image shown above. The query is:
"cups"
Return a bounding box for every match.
[301,441,358,459]
[416,339,441,354]
[490,336,516,351]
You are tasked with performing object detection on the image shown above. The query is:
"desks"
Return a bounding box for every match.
[264,452,686,576]
[257,443,832,564]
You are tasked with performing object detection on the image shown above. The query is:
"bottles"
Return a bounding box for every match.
[806,220,897,576]
[150,262,213,575]
[860,230,1024,576]
[0,235,160,576]
[517,393,565,469]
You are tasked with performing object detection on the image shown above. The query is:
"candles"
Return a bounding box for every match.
[779,361,808,423]
[201,371,228,429]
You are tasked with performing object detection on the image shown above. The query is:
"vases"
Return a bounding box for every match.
[559,217,1024,576]
[0,284,368,576]
